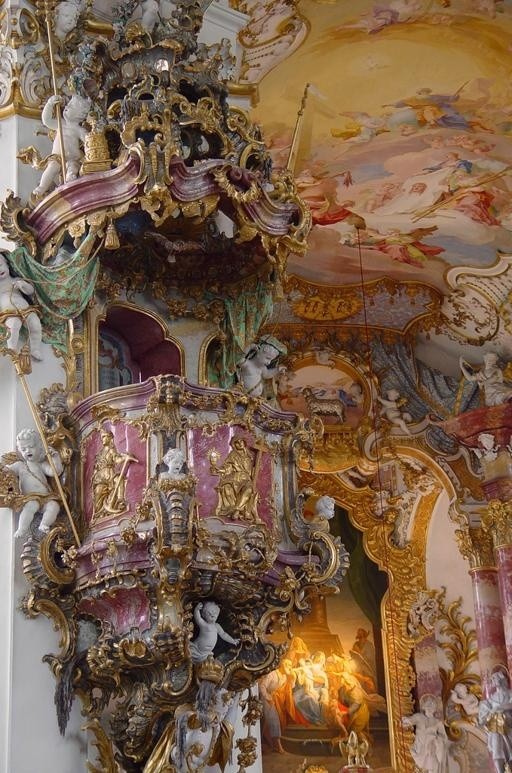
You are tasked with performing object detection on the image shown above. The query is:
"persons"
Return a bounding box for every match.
[90,430,127,524]
[0,254,44,363]
[236,342,288,397]
[52,2,79,41]
[209,434,256,521]
[32,94,92,196]
[158,448,187,482]
[314,495,336,519]
[0,428,63,539]
